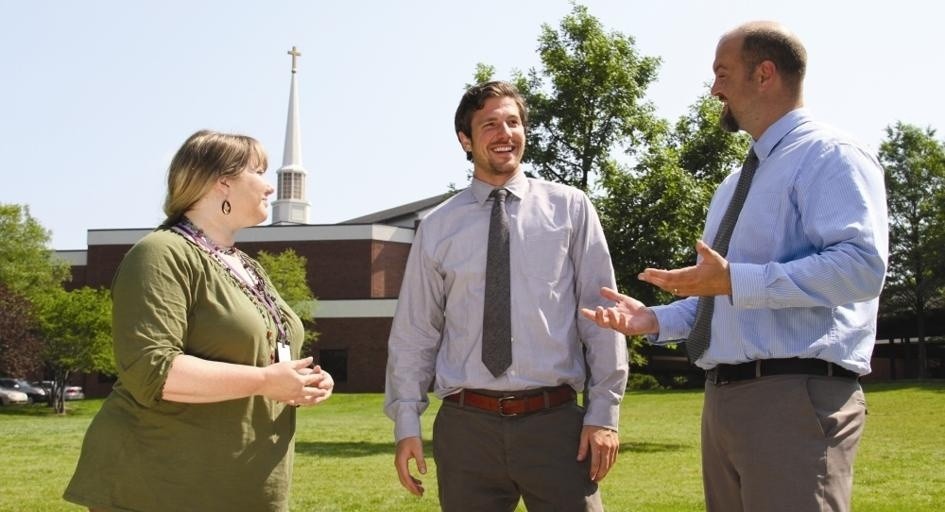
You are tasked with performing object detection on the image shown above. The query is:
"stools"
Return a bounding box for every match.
[446,389,573,416]
[705,358,856,383]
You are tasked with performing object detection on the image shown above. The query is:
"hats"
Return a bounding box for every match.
[0,378,85,405]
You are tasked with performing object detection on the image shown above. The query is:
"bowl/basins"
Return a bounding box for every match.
[180,217,290,360]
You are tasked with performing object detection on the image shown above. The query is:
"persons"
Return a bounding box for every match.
[383,82,630,512]
[63,130,334,512]
[581,21,889,512]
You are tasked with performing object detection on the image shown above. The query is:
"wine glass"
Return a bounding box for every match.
[672,288,678,296]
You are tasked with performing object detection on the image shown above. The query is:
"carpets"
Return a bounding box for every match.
[480,190,510,376]
[684,149,758,363]
